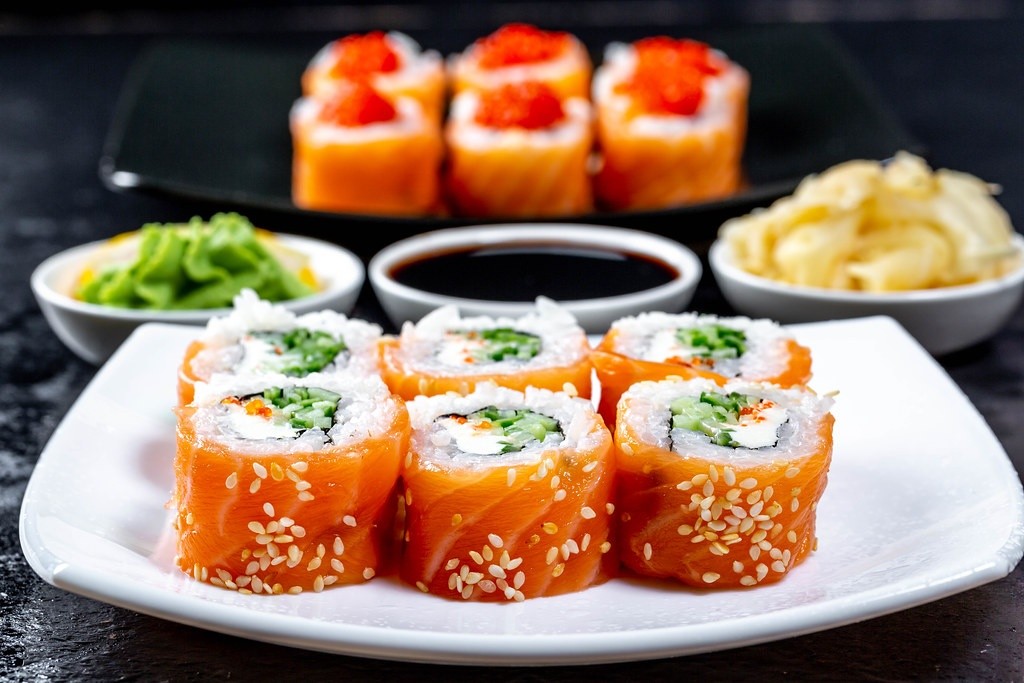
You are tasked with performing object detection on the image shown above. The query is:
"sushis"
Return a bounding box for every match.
[289,23,752,215]
[174,287,834,601]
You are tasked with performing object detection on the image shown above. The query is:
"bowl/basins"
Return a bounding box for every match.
[708,229,1024,355]
[33,225,367,365]
[367,223,702,335]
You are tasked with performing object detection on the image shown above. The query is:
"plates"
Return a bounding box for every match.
[15,316,1024,673]
[97,44,812,238]
